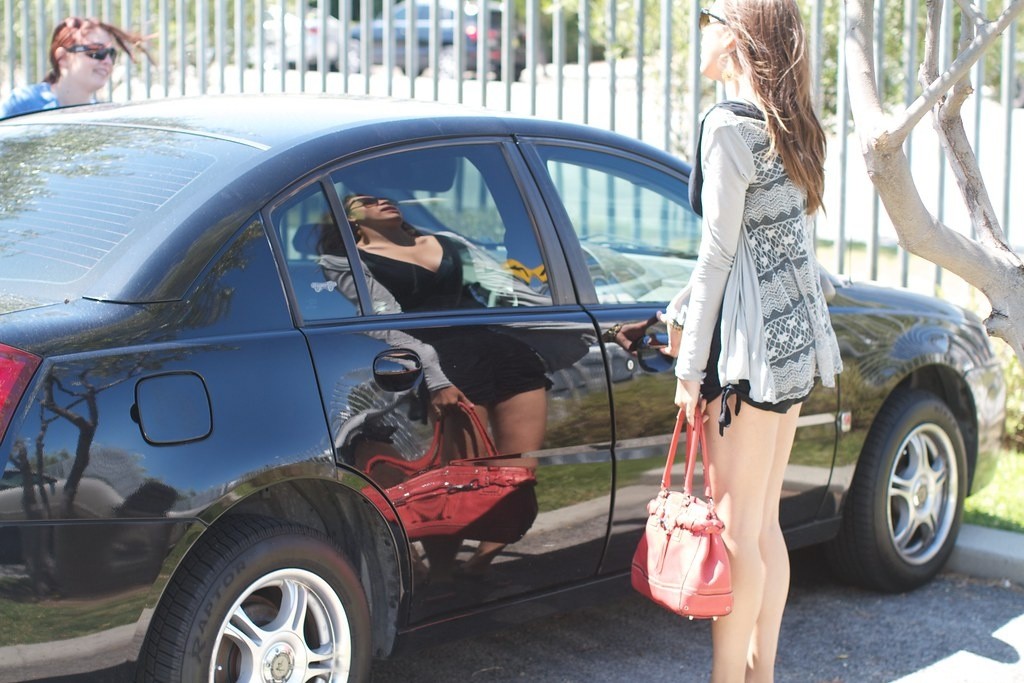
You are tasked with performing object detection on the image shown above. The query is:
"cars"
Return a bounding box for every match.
[243,0,525,81]
[0,91,1007,683]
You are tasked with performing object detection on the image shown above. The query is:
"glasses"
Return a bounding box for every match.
[699,9,726,31]
[67,41,117,64]
[348,196,398,214]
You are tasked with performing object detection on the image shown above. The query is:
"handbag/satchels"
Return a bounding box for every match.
[362,398,539,544]
[631,406,733,620]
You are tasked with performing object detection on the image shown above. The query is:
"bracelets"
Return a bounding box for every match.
[668,317,682,332]
[607,325,621,342]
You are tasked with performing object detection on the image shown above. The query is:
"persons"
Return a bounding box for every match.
[633,0,843,683]
[0,22,157,119]
[318,194,658,593]
